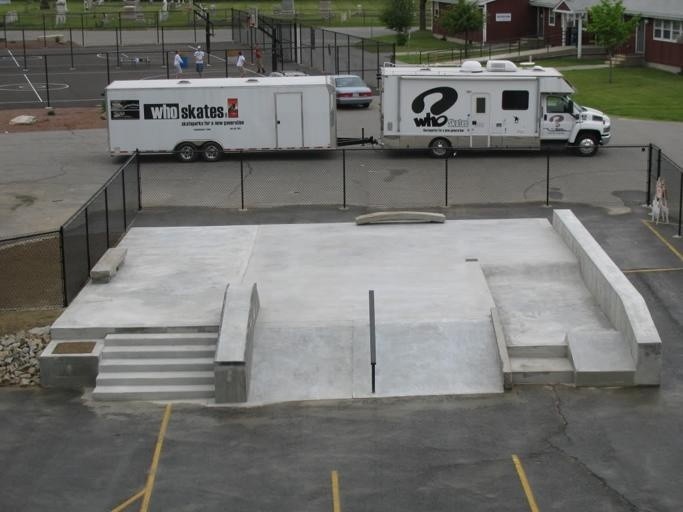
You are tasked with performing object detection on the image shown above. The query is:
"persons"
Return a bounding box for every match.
[193,44,205,79]
[253,41,266,73]
[173,48,184,78]
[235,51,251,77]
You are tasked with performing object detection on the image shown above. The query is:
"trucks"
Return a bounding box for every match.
[377,55,613,161]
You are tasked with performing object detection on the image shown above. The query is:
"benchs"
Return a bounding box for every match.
[90,247,127,283]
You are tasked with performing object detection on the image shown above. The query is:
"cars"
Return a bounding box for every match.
[330,74,374,108]
